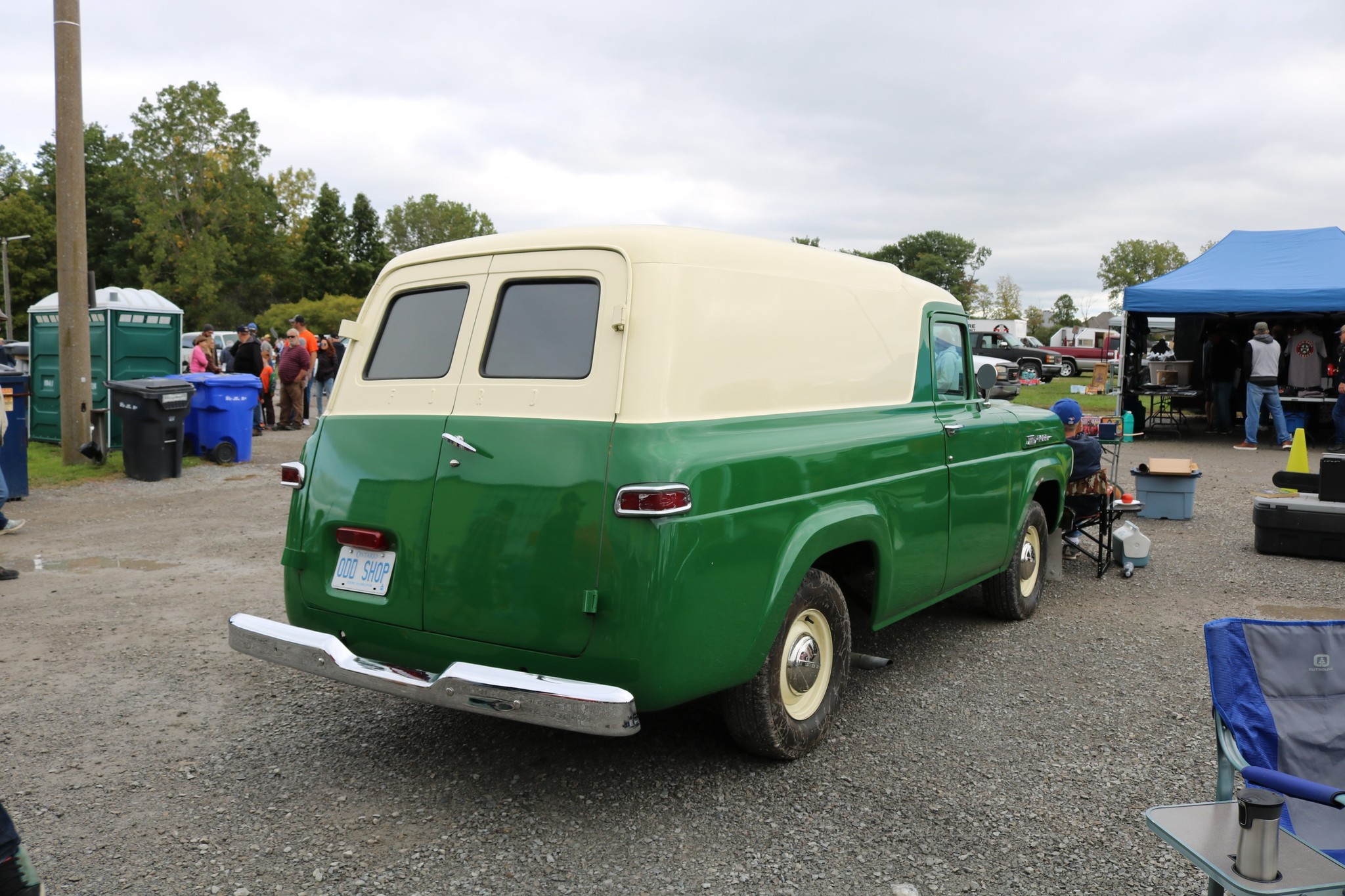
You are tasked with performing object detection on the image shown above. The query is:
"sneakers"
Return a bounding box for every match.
[1233,442,1258,450]
[1282,441,1293,449]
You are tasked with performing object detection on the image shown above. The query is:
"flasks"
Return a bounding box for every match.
[1236,788,1284,881]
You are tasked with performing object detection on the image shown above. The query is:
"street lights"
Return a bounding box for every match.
[1,235,32,340]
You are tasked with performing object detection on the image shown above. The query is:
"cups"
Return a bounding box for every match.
[1327,363,1334,375]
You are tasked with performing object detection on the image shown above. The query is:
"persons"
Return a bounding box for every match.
[933,328,960,391]
[0,338,26,581]
[1232,322,1345,453]
[1049,398,1103,560]
[190,314,345,436]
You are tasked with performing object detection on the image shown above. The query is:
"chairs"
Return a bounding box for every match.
[1055,468,1143,576]
[981,340,987,348]
[1145,613,1345,896]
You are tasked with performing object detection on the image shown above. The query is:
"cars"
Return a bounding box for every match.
[969,330,1063,385]
[935,353,1022,402]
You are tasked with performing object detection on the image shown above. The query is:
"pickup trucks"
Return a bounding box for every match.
[1039,335,1136,378]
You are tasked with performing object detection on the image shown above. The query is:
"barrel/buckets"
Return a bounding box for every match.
[1121,410,1134,442]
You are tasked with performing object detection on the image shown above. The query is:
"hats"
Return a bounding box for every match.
[330,333,339,339]
[261,350,270,362]
[202,323,213,332]
[248,323,257,330]
[289,314,304,322]
[1254,322,1268,332]
[1334,325,1345,333]
[195,335,207,345]
[1050,397,1085,424]
[233,324,248,331]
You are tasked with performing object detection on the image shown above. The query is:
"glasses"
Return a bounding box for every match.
[287,336,295,338]
[1339,332,1345,336]
[267,337,270,339]
[320,343,327,346]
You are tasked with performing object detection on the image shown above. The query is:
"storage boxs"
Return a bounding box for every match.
[1252,491,1345,561]
[1149,457,1199,475]
[1130,467,1202,520]
[1148,360,1194,385]
[1283,411,1310,432]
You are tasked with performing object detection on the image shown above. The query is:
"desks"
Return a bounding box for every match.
[1088,435,1124,511]
[1269,396,1338,447]
[1133,382,1205,434]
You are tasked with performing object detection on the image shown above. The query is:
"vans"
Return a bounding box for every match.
[229,226,1074,767]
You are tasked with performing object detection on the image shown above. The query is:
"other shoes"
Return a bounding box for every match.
[263,424,276,429]
[1219,427,1232,434]
[1327,443,1345,453]
[1061,542,1081,560]
[285,423,301,430]
[302,419,310,426]
[1204,423,1218,433]
[252,428,262,436]
[272,424,288,431]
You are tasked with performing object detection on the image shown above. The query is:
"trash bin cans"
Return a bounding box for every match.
[0,364,29,501]
[102,378,196,483]
[150,372,263,465]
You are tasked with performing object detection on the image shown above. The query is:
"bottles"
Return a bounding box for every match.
[1123,561,1134,577]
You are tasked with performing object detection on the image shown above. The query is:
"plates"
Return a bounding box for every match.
[1114,499,1140,506]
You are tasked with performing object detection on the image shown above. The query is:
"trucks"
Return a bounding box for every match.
[966,319,1046,348]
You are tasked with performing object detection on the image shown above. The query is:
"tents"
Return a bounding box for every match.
[1106,315,1175,364]
[1117,226,1345,415]
